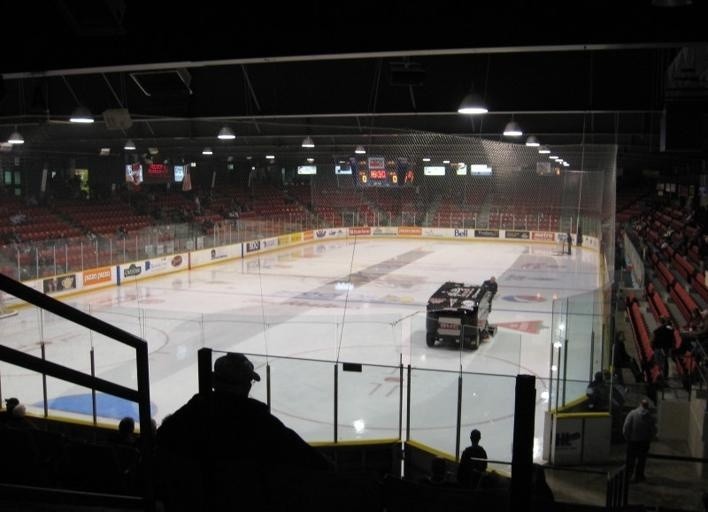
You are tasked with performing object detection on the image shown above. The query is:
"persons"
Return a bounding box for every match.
[123,353,386,512]
[584,178,707,484]
[532,463,558,512]
[482,276,498,313]
[1,177,544,282]
[2,399,40,430]
[460,429,488,472]
[108,418,137,445]
[567,231,572,255]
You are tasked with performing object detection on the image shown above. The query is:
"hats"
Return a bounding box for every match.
[215,354,260,386]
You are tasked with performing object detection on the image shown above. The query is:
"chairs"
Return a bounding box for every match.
[285,183,604,242]
[613,183,706,397]
[0,182,331,286]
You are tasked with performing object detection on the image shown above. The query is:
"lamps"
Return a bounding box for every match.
[422,157,468,167]
[301,136,316,148]
[66,104,96,124]
[538,147,569,169]
[524,130,540,147]
[201,145,215,156]
[215,124,240,141]
[8,129,26,145]
[123,140,136,151]
[189,161,197,166]
[457,91,489,118]
[306,158,316,163]
[264,154,276,160]
[353,145,366,154]
[501,116,522,137]
